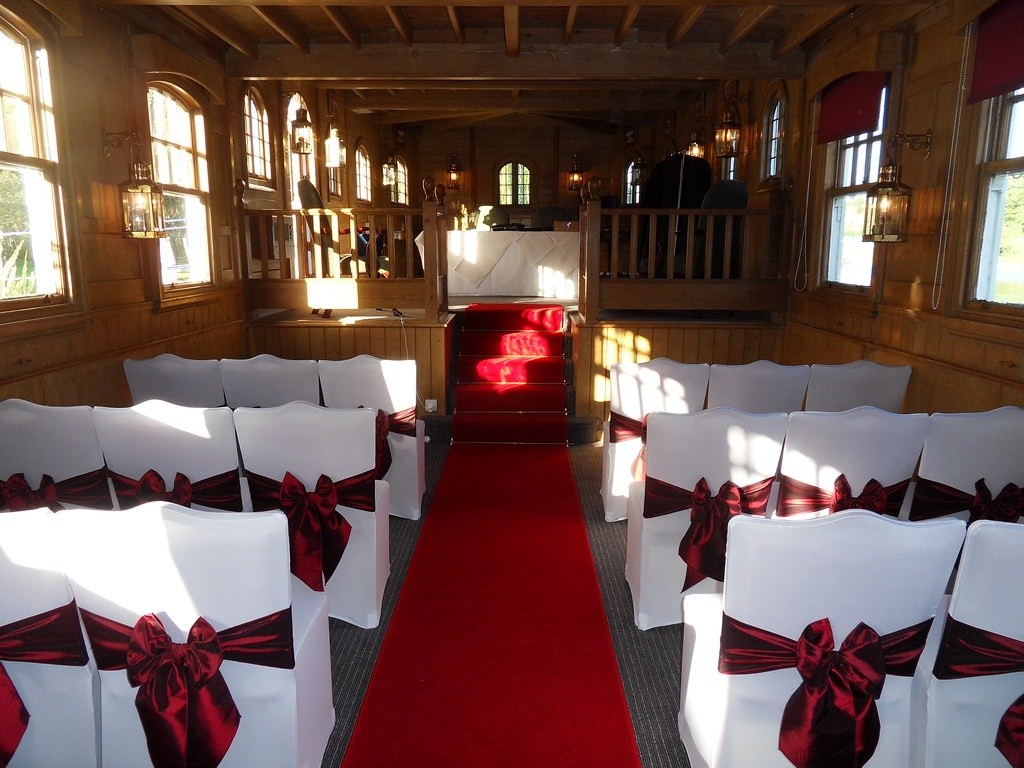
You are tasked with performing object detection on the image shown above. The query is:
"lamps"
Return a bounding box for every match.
[446,152,461,189]
[382,147,396,186]
[860,128,933,242]
[713,93,750,157]
[631,154,648,186]
[685,130,705,159]
[283,89,312,154]
[567,152,584,191]
[101,127,166,239]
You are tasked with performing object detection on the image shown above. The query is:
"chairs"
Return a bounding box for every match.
[600,356,710,521]
[297,178,403,317]
[707,359,809,413]
[60,502,337,768]
[318,355,425,520]
[221,353,324,409]
[1,397,121,510]
[678,509,966,768]
[909,517,1023,768]
[599,154,752,279]
[0,508,102,767]
[470,203,510,230]
[92,399,253,511]
[765,405,929,520]
[805,359,912,414]
[899,406,1024,523]
[624,406,789,630]
[123,353,225,406]
[234,400,389,628]
